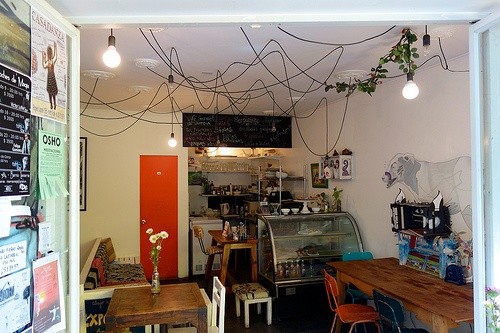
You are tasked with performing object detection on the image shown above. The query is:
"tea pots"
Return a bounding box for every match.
[220,203,229,215]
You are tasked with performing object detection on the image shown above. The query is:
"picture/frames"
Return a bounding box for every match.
[80,136,87,212]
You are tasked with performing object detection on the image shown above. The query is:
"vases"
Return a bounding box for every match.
[151,266,160,293]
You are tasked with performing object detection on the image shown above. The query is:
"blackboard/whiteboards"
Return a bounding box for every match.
[183,113,292,148]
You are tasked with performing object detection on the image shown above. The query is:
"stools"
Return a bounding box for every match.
[232,284,273,328]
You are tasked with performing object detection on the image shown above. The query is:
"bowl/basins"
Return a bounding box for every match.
[281,209,290,215]
[291,209,299,214]
[264,172,275,178]
[312,207,321,213]
[275,171,288,179]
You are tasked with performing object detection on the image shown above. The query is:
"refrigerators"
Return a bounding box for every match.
[189,216,223,276]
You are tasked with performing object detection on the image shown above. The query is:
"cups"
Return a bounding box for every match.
[201,161,250,171]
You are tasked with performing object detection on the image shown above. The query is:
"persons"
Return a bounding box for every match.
[22,118,32,171]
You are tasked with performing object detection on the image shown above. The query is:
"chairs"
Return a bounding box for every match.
[192,225,232,286]
[322,269,430,333]
[168,275,225,333]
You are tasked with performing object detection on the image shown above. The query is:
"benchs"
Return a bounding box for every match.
[81,237,152,333]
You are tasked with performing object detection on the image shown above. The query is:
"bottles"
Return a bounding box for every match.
[236,205,239,214]
[240,207,244,215]
[277,260,306,278]
[208,180,214,193]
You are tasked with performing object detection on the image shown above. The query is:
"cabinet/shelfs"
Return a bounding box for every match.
[188,156,306,217]
[257,212,364,322]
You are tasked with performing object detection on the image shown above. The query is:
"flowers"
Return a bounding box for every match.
[140,219,169,289]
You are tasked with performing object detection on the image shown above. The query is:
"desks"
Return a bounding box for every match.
[325,257,474,333]
[204,230,258,286]
